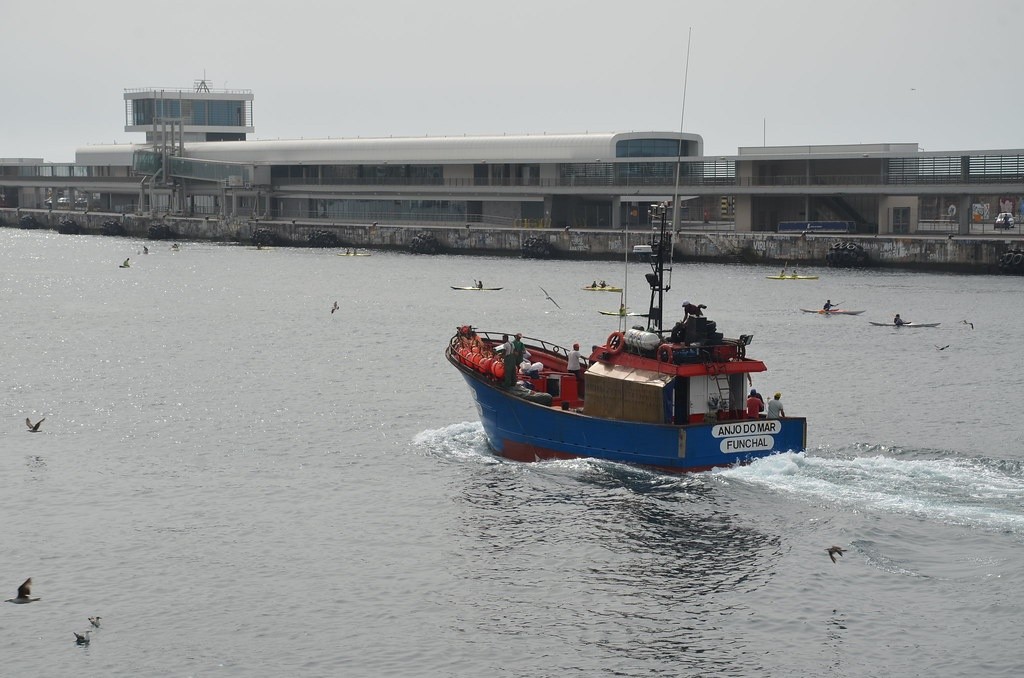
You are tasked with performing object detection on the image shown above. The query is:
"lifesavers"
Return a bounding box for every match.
[656,344,673,364]
[606,331,624,354]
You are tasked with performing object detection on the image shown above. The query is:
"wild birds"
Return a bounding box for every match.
[331,300,339,314]
[5,578,40,605]
[538,285,562,310]
[957,320,975,329]
[826,546,847,563]
[26,418,44,433]
[119,258,131,268]
[935,345,949,351]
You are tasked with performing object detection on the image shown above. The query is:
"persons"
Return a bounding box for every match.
[779,270,784,277]
[258,243,261,250]
[592,281,597,288]
[767,392,785,419]
[619,303,626,314]
[704,209,711,223]
[477,281,483,289]
[1002,214,1009,230]
[823,300,838,310]
[747,389,764,419]
[894,314,904,325]
[511,332,526,372]
[346,249,350,254]
[600,280,609,288]
[792,269,798,277]
[568,343,591,402]
[493,335,517,386]
[681,300,703,323]
[561,401,570,411]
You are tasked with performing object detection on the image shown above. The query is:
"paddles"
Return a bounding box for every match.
[473,278,478,288]
[819,299,847,314]
[794,263,798,280]
[784,262,788,280]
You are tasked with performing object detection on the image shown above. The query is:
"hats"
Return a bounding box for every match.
[774,392,781,398]
[516,333,523,338]
[750,389,756,395]
[573,343,580,348]
[682,300,690,307]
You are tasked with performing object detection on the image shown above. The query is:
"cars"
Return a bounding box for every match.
[44,197,87,207]
[994,213,1014,229]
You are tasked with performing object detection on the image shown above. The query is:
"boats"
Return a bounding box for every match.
[119,265,129,269]
[450,286,504,292]
[766,275,820,279]
[246,247,273,250]
[445,29,809,479]
[137,250,148,255]
[598,310,648,318]
[169,247,180,252]
[337,252,370,256]
[800,308,867,316]
[870,321,942,328]
[581,287,624,293]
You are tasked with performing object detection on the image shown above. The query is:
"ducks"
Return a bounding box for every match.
[89,616,102,627]
[73,630,92,644]
